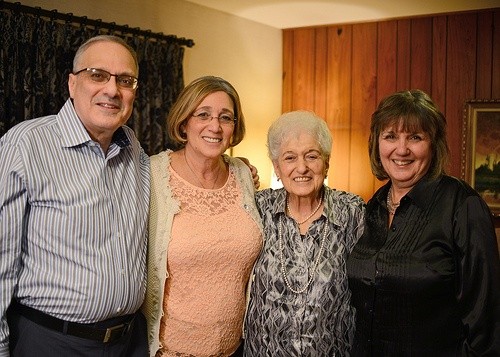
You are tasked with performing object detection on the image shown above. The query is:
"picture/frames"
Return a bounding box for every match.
[460,99,500,218]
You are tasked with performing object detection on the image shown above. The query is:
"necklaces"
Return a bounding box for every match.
[279,187,330,294]
[386,186,403,216]
[183,149,222,190]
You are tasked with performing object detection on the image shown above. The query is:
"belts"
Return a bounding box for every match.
[11,298,142,343]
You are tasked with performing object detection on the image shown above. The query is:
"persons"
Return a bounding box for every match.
[0,34,259,357]
[137,75,266,357]
[346,89,500,357]
[239,110,367,357]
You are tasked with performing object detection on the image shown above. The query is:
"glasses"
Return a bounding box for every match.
[74,66,140,91]
[192,110,237,126]
